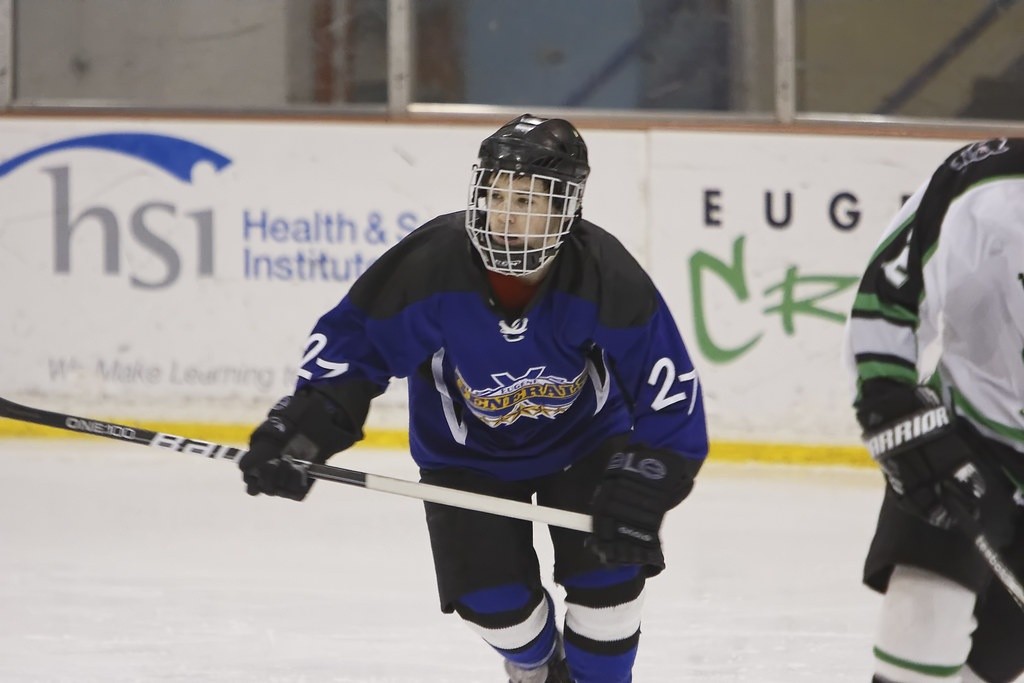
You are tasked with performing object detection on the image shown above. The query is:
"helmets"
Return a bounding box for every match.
[464,115,590,277]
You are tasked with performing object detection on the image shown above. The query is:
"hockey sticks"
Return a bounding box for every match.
[0,394,598,536]
[963,521,1024,609]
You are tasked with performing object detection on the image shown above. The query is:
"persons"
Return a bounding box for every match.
[848,136,1024,683]
[239,113,709,683]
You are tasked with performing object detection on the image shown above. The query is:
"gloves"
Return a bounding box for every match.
[238,395,365,501]
[580,447,693,566]
[855,380,992,530]
